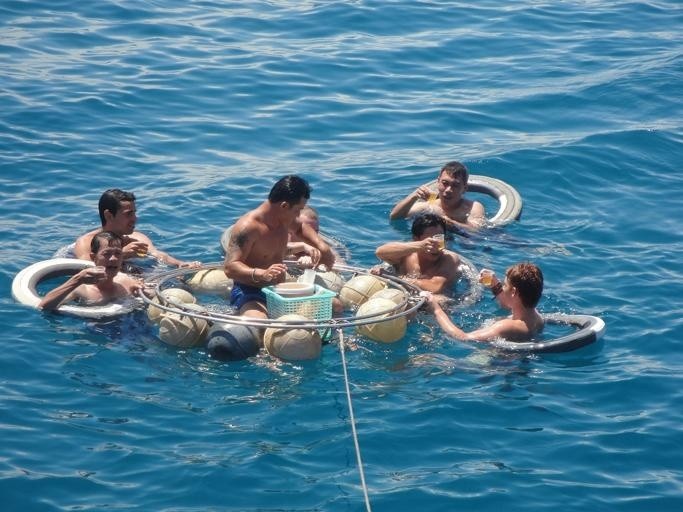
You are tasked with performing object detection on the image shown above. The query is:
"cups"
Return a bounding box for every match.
[427,188,439,203]
[136,241,149,259]
[433,233,446,252]
[481,270,495,288]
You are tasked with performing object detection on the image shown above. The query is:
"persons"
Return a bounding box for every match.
[285,206,350,267]
[33,230,154,326]
[74,187,200,269]
[388,260,544,375]
[370,212,462,306]
[220,173,336,322]
[388,161,484,237]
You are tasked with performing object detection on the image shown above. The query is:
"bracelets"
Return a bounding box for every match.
[489,282,503,295]
[251,268,260,284]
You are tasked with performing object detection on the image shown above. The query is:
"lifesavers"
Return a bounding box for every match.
[484,311,605,357]
[380,250,484,314]
[411,175,522,237]
[10,257,149,321]
[220,221,353,264]
[55,242,167,283]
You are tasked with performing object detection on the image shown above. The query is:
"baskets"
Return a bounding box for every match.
[259,281,338,345]
